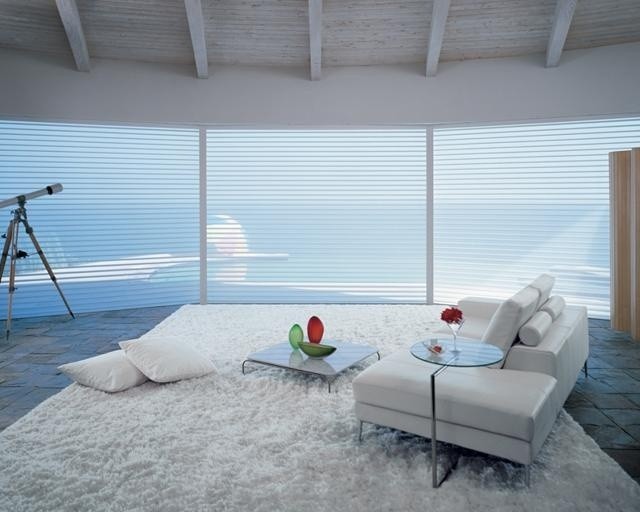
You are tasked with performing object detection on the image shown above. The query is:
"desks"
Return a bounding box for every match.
[409,337,505,487]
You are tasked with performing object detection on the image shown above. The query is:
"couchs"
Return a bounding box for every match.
[352,274,592,488]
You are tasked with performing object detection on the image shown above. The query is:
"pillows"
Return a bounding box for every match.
[55,333,219,395]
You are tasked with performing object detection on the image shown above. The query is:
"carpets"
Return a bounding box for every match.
[0,302,639,512]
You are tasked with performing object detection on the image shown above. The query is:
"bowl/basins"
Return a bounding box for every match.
[297,341,336,360]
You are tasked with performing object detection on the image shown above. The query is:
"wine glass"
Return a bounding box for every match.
[446,318,466,352]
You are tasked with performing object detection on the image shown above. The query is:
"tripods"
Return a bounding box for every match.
[0,201,76,340]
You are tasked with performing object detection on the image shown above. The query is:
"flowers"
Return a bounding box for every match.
[440,305,464,327]
